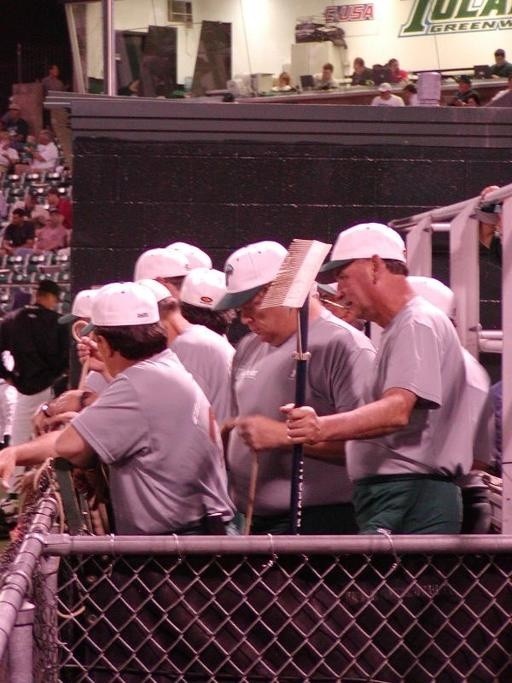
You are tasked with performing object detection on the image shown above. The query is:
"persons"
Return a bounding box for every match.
[351,57,374,83]
[449,73,481,108]
[2,188,71,255]
[384,57,412,83]
[402,84,419,105]
[2,222,490,582]
[489,48,511,75]
[272,71,290,90]
[313,63,339,87]
[484,76,512,105]
[370,82,405,107]
[1,63,76,182]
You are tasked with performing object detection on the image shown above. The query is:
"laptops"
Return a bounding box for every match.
[300,76,323,90]
[475,65,494,79]
[373,70,390,84]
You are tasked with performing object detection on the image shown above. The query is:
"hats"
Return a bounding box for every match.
[33,280,59,292]
[59,222,454,335]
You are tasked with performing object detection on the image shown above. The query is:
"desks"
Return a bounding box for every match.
[218,76,511,105]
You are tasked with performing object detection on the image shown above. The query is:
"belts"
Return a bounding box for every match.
[355,473,449,483]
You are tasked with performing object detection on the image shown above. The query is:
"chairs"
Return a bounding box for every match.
[1,168,72,324]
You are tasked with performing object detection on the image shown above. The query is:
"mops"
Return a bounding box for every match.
[259,235,334,533]
